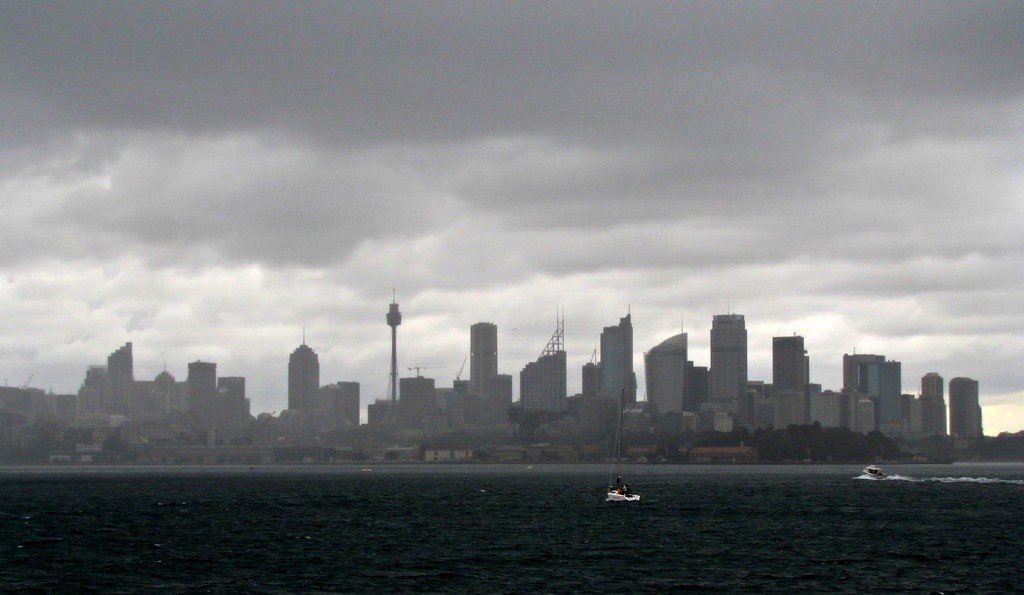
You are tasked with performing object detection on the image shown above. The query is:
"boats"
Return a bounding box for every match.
[863,464,884,476]
[602,389,641,502]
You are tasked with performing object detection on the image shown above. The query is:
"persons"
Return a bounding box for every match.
[614,475,633,499]
